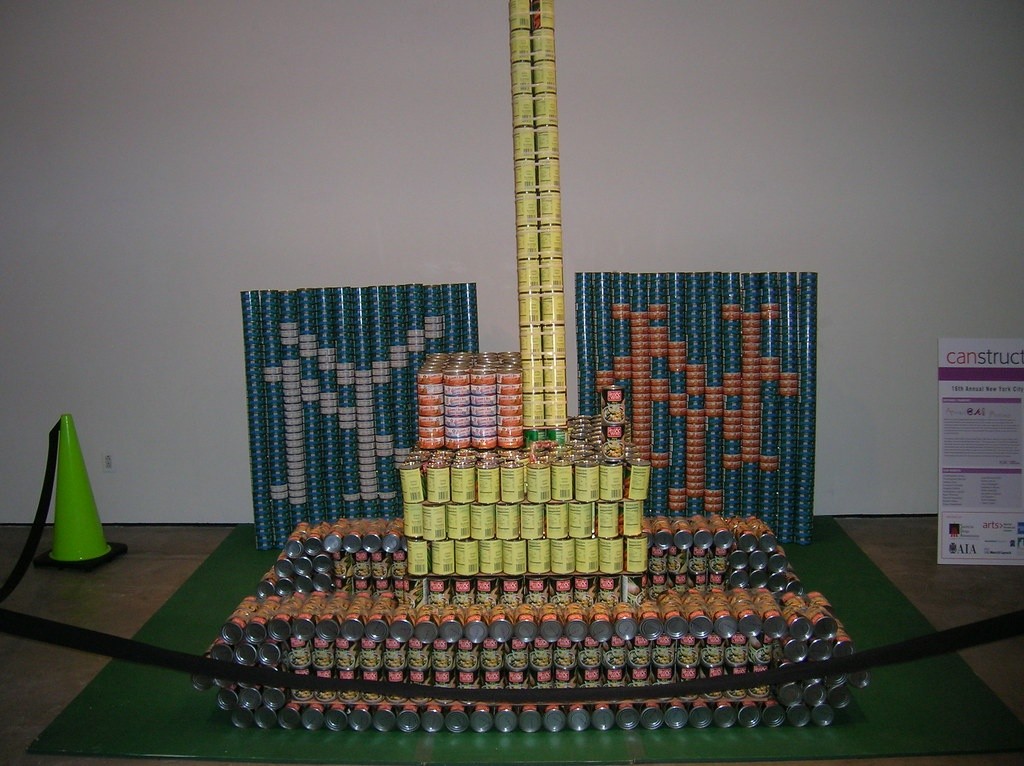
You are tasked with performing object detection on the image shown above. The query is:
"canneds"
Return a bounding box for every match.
[191,272,873,735]
[507,0,566,292]
